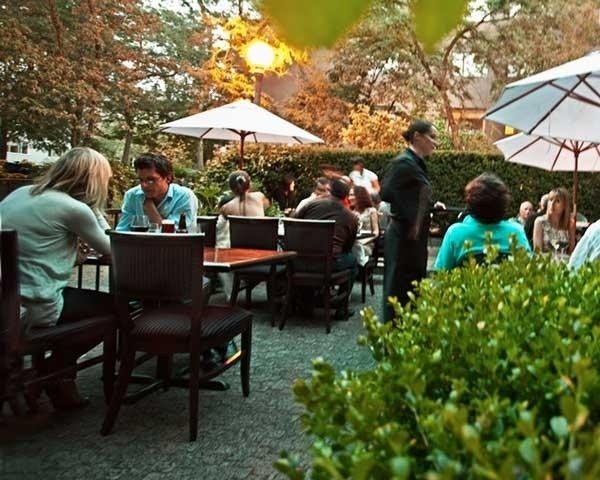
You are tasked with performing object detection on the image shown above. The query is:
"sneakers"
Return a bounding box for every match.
[34,360,91,413]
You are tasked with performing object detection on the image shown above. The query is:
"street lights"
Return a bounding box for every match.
[245,39,277,104]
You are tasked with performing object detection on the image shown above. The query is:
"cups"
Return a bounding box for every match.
[130,215,150,233]
[149,224,159,233]
[162,220,175,233]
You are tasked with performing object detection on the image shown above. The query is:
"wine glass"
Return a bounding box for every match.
[550,230,569,260]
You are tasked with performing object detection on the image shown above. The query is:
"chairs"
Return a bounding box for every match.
[457,246,513,269]
[426,206,468,247]
[0,210,381,442]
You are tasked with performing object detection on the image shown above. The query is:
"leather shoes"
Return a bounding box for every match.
[331,307,358,321]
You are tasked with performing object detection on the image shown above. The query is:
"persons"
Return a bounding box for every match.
[438,173,530,277]
[0,147,120,418]
[380,122,448,329]
[117,155,201,304]
[220,155,388,323]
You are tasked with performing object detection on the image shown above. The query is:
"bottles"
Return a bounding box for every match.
[177,215,188,233]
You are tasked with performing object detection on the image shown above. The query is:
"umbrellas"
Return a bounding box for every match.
[158,98,324,199]
[482,51,600,273]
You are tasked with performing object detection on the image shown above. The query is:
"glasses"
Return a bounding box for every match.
[135,176,164,186]
